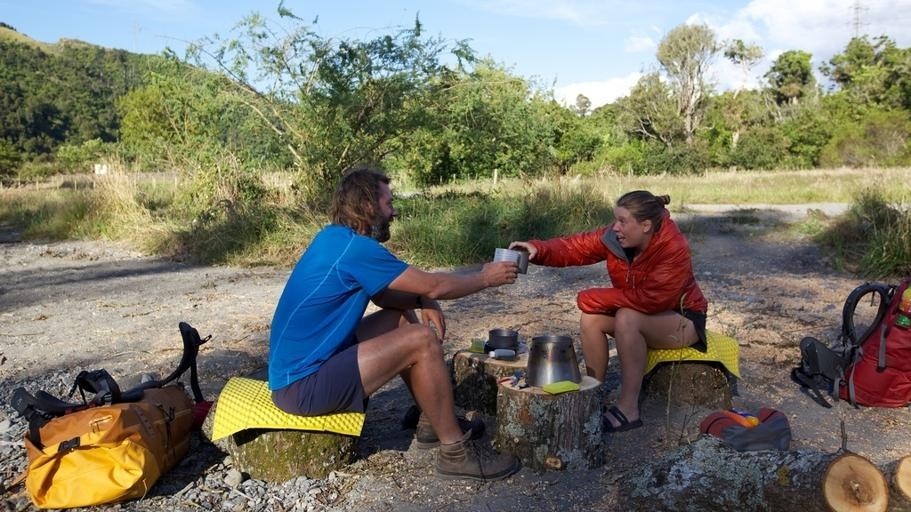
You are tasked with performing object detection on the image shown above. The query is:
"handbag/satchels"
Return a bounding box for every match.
[700,404,792,452]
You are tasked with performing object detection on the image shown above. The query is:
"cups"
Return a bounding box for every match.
[494,248,530,277]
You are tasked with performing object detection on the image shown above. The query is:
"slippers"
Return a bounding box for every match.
[602,403,644,433]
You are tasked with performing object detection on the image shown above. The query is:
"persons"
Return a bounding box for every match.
[508,190,708,433]
[267,169,522,481]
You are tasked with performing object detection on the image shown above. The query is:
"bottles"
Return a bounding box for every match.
[488,349,516,360]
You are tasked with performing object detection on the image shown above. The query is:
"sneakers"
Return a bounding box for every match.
[436,429,522,482]
[416,412,487,450]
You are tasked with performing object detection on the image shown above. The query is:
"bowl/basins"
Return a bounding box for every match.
[489,329,518,348]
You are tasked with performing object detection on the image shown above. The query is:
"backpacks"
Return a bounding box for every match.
[833,277,911,412]
